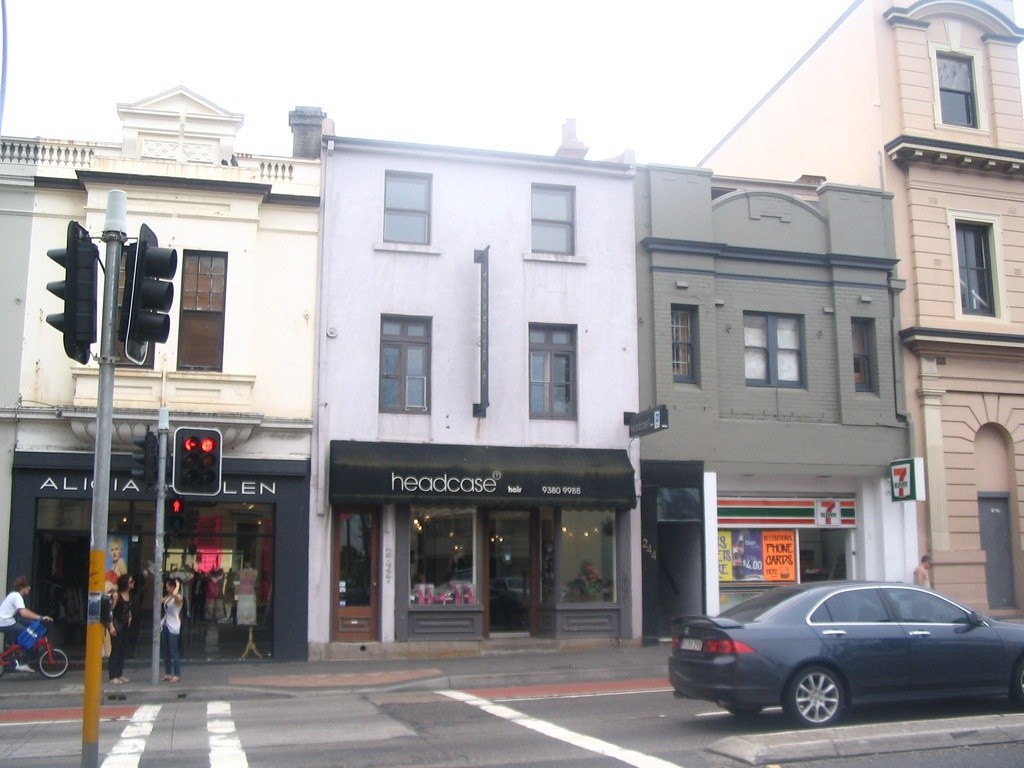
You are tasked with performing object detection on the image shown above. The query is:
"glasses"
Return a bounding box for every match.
[127,578,134,584]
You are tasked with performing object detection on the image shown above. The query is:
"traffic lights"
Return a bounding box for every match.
[45,220,103,365]
[118,222,177,366]
[172,426,225,498]
[130,430,155,489]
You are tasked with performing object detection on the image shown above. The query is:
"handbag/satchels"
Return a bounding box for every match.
[17,617,47,650]
[102,627,112,658]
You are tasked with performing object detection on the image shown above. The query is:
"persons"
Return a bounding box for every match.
[159,576,183,684]
[104,535,128,658]
[0,575,55,673]
[105,574,136,685]
[169,562,260,626]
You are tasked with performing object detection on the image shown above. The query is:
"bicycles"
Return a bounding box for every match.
[0,619,71,681]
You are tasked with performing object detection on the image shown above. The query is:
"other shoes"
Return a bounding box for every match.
[160,678,180,683]
[109,676,129,685]
[15,660,35,672]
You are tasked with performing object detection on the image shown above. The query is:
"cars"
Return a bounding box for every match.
[668,579,1023,731]
[490,576,532,598]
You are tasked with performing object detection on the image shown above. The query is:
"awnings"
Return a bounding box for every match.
[328,439,638,513]
